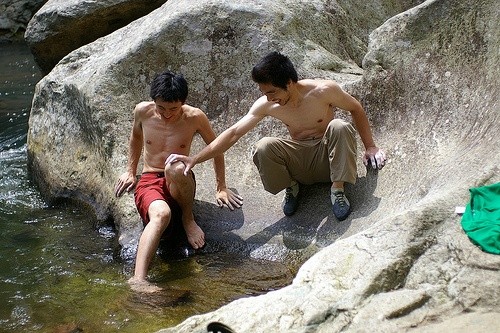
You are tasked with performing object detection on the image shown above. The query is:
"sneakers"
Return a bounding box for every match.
[282,182,303,216]
[330,186,351,221]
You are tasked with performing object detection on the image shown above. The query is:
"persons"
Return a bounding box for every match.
[164,51,388,221]
[113,72,245,280]
